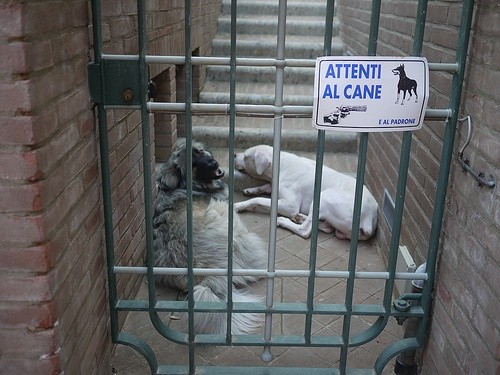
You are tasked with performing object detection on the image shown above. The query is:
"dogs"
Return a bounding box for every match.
[146,139,268,334]
[232,144,380,242]
